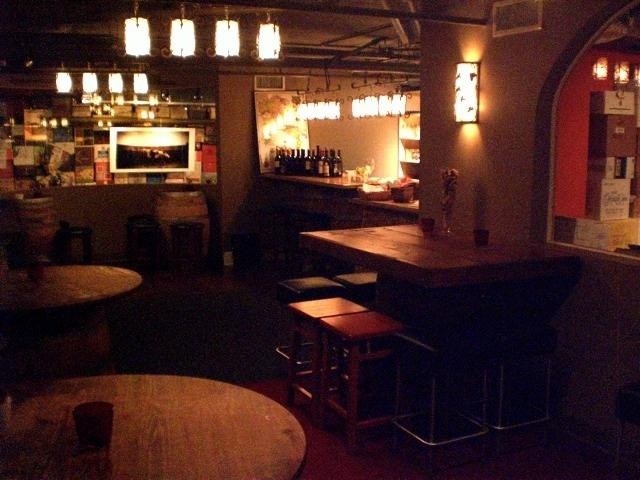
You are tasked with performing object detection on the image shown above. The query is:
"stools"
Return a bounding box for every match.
[278,270,564,473]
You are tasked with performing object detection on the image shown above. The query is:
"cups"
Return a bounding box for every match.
[473,228,490,246]
[421,217,435,233]
[70,400,114,449]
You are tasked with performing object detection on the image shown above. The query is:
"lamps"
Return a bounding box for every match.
[169,1,195,58]
[125,1,151,57]
[82,60,99,94]
[293,59,409,119]
[90,93,159,119]
[55,56,73,94]
[132,61,150,94]
[108,60,124,94]
[453,61,480,124]
[215,1,240,59]
[256,1,281,61]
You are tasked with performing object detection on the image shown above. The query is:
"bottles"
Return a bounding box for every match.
[274,144,344,177]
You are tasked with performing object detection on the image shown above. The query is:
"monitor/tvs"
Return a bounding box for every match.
[109,127,196,173]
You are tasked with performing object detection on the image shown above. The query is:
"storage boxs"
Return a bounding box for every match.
[585,91,640,222]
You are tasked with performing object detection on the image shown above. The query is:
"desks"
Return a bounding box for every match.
[1,265,149,376]
[1,372,308,478]
[297,221,580,447]
